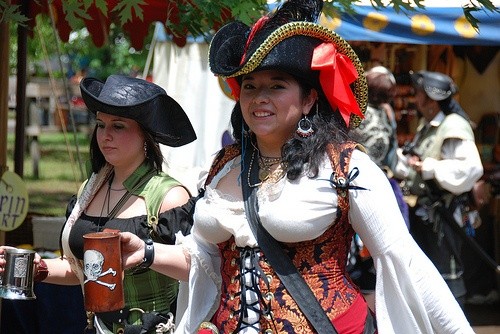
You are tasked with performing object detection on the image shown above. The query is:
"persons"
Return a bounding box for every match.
[0,74,197,334]
[118,0,476,334]
[346,66,411,314]
[394,71,484,316]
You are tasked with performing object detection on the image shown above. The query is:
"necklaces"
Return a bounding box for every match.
[97,168,158,232]
[247,143,288,187]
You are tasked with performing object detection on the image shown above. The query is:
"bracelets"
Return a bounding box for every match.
[33,259,49,283]
[135,238,155,268]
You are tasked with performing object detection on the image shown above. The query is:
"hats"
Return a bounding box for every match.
[81,73,197,148]
[209,0,368,132]
[410,70,457,100]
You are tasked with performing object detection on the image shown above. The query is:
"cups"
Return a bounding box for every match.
[83,229,125,312]
[0,245,36,300]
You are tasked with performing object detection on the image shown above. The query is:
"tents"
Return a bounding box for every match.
[142,0,500,197]
